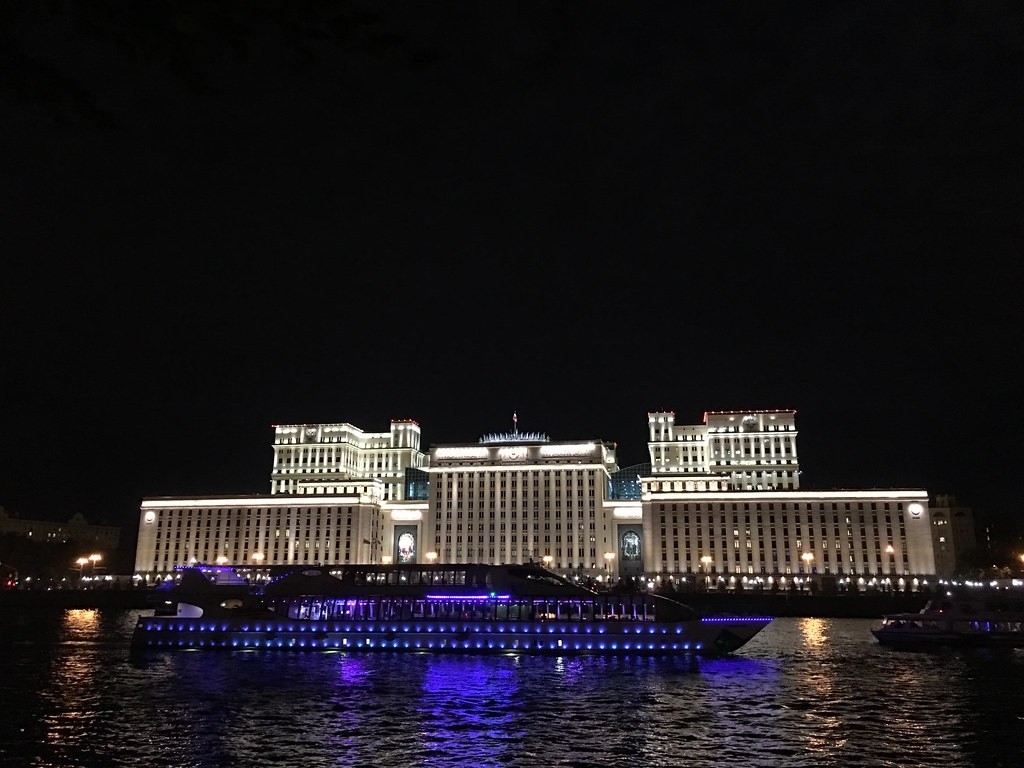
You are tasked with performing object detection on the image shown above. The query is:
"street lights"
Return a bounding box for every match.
[77,553,102,578]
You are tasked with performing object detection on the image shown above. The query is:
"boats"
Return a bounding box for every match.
[132,560,778,657]
[870,586,1023,655]
